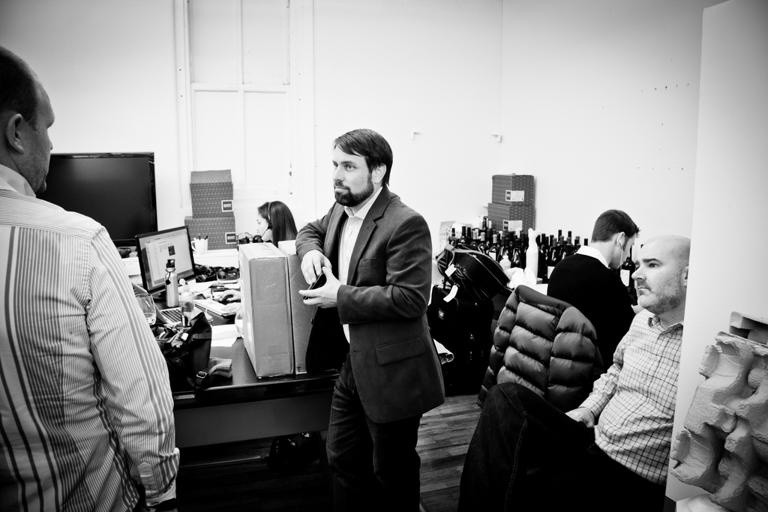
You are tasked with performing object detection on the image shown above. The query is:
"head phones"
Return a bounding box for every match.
[251,200,275,243]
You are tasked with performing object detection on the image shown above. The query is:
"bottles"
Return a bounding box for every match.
[164,258,178,307]
[447,216,636,296]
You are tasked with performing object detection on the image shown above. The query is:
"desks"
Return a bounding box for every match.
[136,280,452,512]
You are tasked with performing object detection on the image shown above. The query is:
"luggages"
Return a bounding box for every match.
[427,285,494,398]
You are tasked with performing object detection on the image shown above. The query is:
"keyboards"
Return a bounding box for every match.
[195,295,233,319]
[158,307,188,328]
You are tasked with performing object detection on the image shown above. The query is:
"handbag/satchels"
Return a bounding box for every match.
[436,243,510,301]
[149,312,211,391]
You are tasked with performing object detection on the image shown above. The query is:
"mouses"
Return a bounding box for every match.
[217,293,236,303]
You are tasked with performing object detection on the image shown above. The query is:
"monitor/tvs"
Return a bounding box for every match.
[135,224,197,302]
[45,152,158,248]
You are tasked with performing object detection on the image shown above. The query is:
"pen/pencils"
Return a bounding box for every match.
[198,235,208,240]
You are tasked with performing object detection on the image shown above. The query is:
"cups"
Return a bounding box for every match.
[135,292,157,325]
[190,238,208,254]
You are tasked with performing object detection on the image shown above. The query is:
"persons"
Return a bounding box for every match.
[294,129,448,512]
[566,233,690,512]
[256,201,298,247]
[0,45,185,512]
[547,210,641,373]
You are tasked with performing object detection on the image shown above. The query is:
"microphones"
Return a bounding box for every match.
[254,225,275,243]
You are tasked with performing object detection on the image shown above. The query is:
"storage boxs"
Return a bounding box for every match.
[190,170,234,218]
[492,175,535,205]
[488,202,536,235]
[185,215,237,250]
